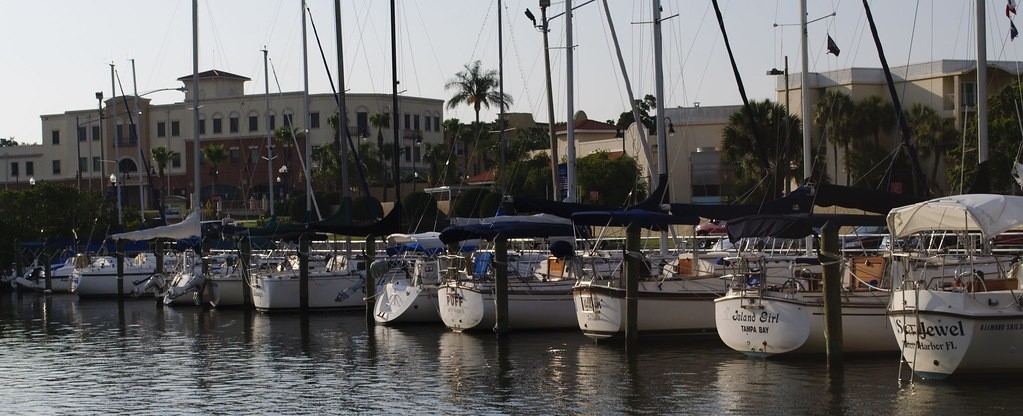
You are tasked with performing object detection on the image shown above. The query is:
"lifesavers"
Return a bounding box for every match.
[370,260,389,278]
[75,254,88,269]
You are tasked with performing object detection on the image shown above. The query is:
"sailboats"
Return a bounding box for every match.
[711,0,1016,361]
[1,0,850,347]
[886,0,1022,385]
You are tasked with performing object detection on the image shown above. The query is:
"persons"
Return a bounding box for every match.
[226,255,233,275]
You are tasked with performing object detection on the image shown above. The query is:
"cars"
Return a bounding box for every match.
[695,219,727,249]
[879,230,980,255]
[839,226,889,258]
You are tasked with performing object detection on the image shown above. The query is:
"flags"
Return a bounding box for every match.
[1006,0,1018,41]
[826,34,840,57]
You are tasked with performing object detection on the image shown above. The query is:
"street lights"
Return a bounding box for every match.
[663,116,677,174]
[615,123,625,153]
[412,130,423,191]
[148,159,156,210]
[109,174,116,211]
[276,176,281,200]
[279,168,286,200]
[123,166,131,208]
[282,165,288,194]
[96,92,105,195]
[766,56,791,196]
